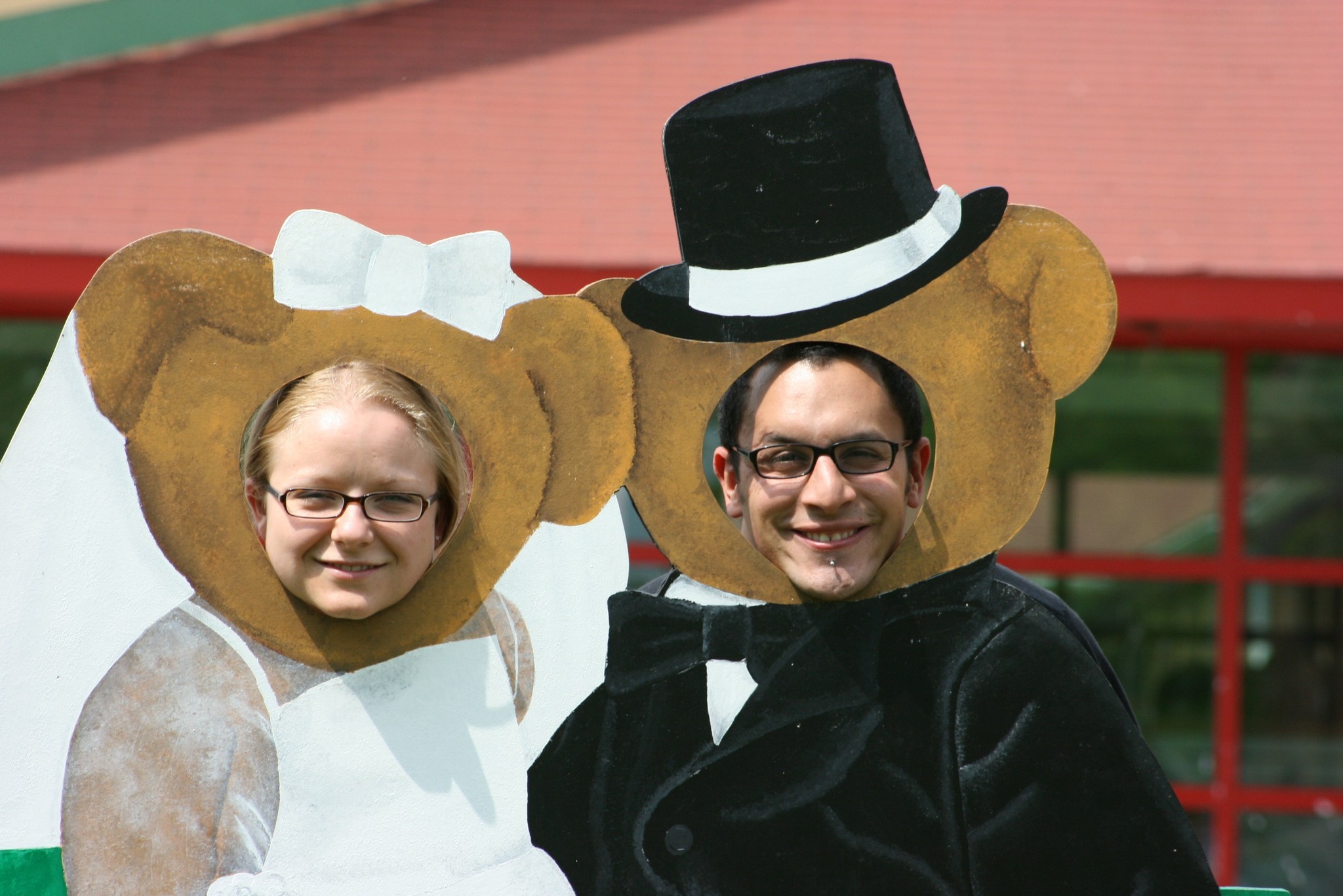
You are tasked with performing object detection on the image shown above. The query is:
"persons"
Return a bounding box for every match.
[713,341,931,607]
[241,361,471,620]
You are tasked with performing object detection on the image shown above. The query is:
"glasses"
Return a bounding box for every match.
[264,481,439,523]
[732,439,913,479]
